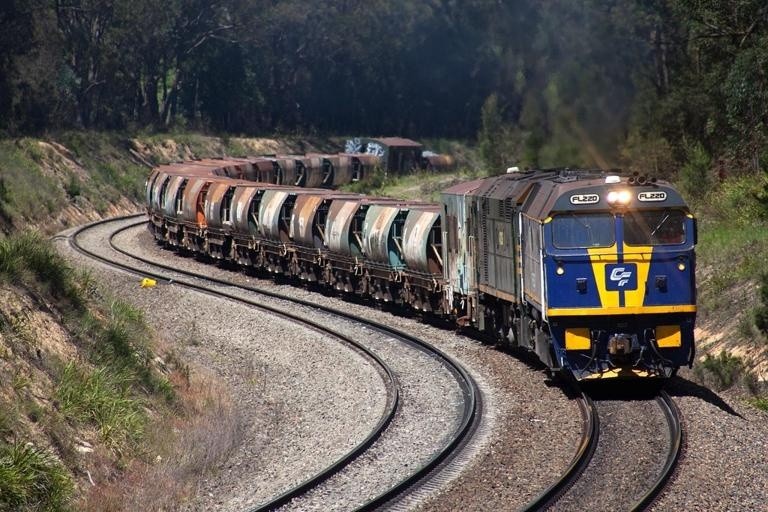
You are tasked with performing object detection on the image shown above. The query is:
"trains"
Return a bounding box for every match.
[145,132,699,386]
